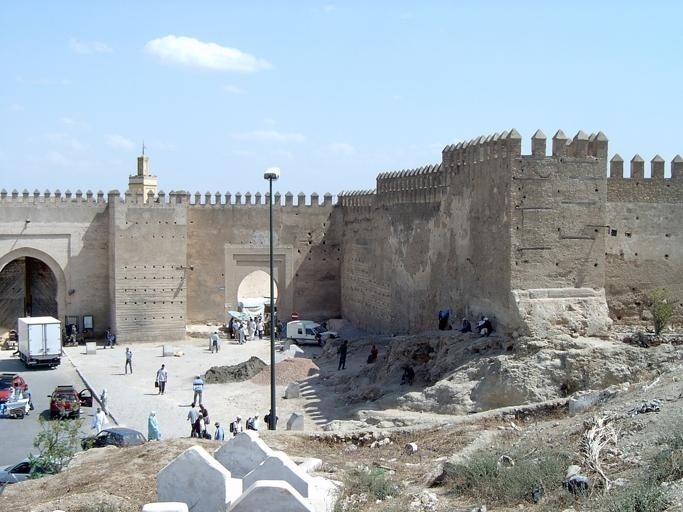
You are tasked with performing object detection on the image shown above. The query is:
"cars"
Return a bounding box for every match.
[80,427,148,452]
[0,327,17,351]
[0,455,68,487]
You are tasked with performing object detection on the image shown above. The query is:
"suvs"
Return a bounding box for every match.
[0,370,28,406]
[46,384,95,418]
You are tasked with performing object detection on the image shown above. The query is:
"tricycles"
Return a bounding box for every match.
[0,385,30,419]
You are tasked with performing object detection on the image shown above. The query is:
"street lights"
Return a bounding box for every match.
[263,164,287,428]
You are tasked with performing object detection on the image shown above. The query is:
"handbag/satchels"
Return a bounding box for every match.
[230,423,233,432]
[155,382,158,387]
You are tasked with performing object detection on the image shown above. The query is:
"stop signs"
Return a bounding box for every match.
[291,312,299,320]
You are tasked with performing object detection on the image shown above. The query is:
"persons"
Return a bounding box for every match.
[67,324,78,342]
[337,340,348,370]
[187,402,278,440]
[100,389,109,414]
[229,311,283,345]
[460,317,473,333]
[13,382,34,410]
[103,327,114,349]
[191,374,203,407]
[91,407,109,436]
[125,347,133,375]
[475,315,493,337]
[211,331,220,353]
[147,411,161,441]
[155,364,168,395]
[400,364,415,385]
[366,345,378,363]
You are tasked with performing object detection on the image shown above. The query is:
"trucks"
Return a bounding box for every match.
[285,320,340,347]
[17,316,64,370]
